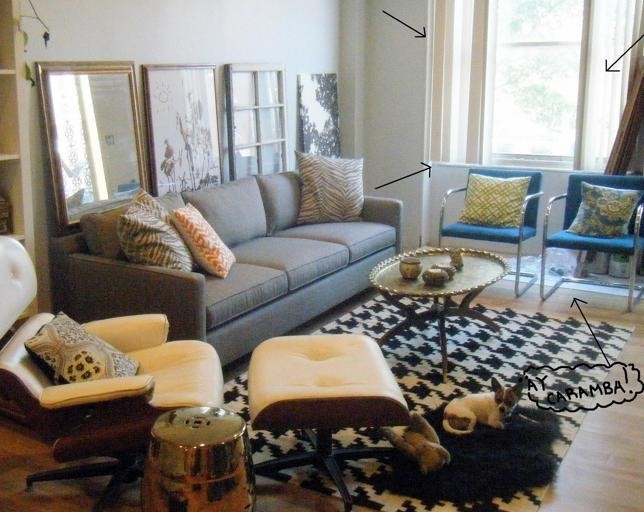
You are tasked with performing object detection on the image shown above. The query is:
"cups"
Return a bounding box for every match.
[398,257,423,280]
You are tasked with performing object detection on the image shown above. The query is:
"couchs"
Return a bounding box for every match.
[44,156,404,376]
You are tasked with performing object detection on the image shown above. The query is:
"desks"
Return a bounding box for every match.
[366,247,512,385]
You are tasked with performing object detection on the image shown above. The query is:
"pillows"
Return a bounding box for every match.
[20,308,142,385]
[456,172,532,230]
[564,178,644,238]
[292,149,366,224]
[115,187,200,273]
[173,202,238,278]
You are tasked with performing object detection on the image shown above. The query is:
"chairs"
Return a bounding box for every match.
[436,166,544,298]
[538,171,644,314]
[3,229,227,511]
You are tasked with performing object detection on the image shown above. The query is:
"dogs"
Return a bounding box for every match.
[442,377,524,436]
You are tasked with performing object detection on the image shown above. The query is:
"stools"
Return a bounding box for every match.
[239,327,413,511]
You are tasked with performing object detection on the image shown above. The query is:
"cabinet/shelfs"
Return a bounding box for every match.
[0,0,37,333]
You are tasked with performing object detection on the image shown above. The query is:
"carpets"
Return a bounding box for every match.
[486,253,644,298]
[206,283,637,512]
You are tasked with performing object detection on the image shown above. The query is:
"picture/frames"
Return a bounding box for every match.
[133,61,227,195]
[33,57,152,240]
